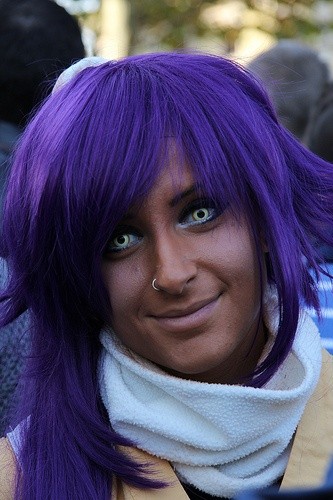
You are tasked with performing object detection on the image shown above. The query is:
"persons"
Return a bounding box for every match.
[247,43,333,355]
[0,51,333,500]
[0,0,123,439]
